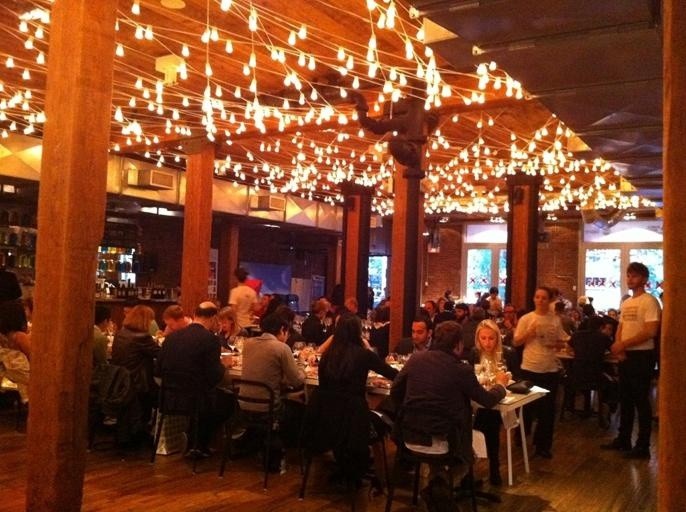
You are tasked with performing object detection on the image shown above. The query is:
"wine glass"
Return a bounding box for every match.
[234,336,244,363]
[227,336,236,354]
[292,342,315,364]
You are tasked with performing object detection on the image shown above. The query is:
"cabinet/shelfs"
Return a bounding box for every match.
[94,246,138,302]
[0,222,38,283]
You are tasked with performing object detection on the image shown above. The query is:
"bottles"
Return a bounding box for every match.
[96,245,133,273]
[495,352,507,374]
[96,279,166,299]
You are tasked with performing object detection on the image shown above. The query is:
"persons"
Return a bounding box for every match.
[228,267,260,339]
[92,285,619,501]
[599,262,662,460]
[0,254,33,404]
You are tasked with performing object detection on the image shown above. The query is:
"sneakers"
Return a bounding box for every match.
[182,436,212,459]
[513,433,527,448]
[459,477,484,492]
[621,446,651,459]
[599,440,632,451]
[597,401,612,432]
[535,447,553,459]
[488,469,503,487]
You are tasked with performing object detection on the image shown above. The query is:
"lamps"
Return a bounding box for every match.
[425,216,448,253]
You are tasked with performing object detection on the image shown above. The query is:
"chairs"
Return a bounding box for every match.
[0,347,30,434]
[220,380,302,486]
[152,368,223,473]
[559,358,602,430]
[87,364,149,450]
[299,390,390,512]
[383,405,478,512]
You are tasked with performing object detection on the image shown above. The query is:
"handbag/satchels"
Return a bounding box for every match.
[505,379,534,394]
[152,407,200,457]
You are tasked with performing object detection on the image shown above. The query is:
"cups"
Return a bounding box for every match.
[221,353,232,370]
[478,376,490,391]
